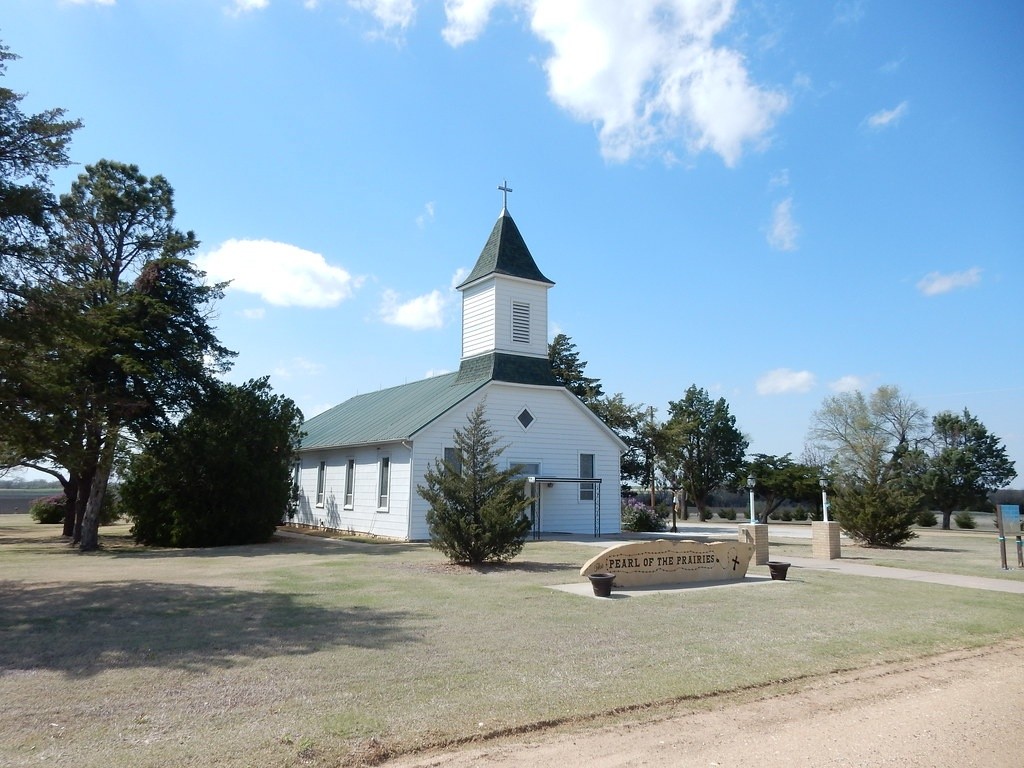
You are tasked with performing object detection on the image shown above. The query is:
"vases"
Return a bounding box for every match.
[766,561,791,580]
[588,573,616,598]
